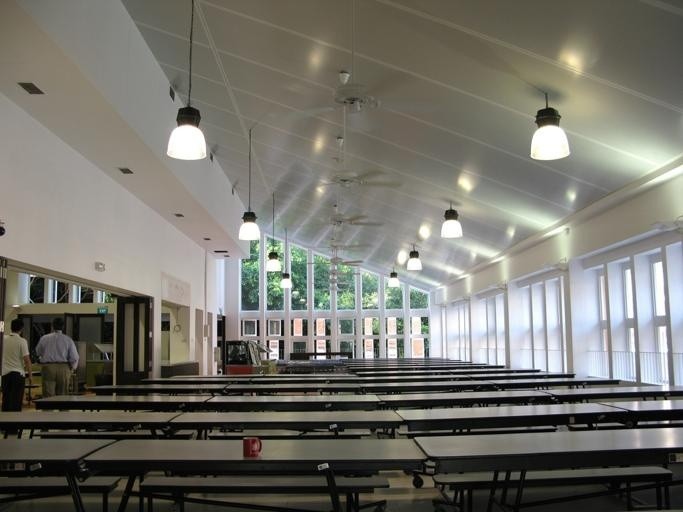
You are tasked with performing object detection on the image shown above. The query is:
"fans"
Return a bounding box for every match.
[292,43,389,295]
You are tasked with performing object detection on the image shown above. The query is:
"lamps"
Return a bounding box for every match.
[280,228,292,288]
[406,242,422,271]
[239,128,260,241]
[530,91,571,161]
[440,201,463,238]
[388,267,400,288]
[167,0,207,160]
[267,191,281,271]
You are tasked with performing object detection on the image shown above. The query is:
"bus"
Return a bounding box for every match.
[217,338,265,375]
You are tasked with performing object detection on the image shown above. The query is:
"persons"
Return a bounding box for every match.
[1,319,32,434]
[35,319,79,412]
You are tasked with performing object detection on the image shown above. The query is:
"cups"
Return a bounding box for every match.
[243,437,263,459]
